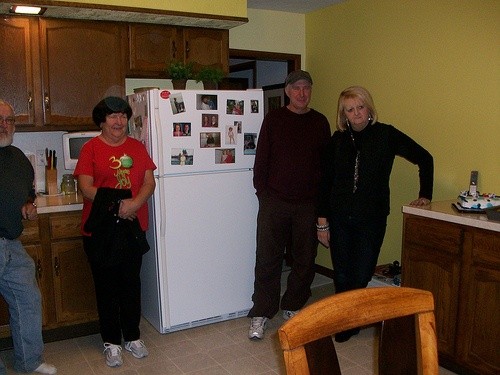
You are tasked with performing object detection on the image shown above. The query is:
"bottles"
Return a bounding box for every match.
[60,174,83,195]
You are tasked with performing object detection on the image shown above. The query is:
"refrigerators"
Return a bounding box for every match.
[128,87,264,334]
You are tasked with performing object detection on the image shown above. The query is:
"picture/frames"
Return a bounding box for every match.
[262,83,287,118]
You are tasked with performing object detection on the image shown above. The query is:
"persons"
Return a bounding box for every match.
[74,96,158,367]
[121,90,266,170]
[0,100,59,374]
[245,68,333,341]
[309,85,434,344]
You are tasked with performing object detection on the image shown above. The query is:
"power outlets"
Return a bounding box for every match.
[36,151,44,167]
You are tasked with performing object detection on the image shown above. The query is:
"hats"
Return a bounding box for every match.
[285,69,312,86]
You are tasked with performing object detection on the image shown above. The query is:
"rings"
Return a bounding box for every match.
[421,199,424,203]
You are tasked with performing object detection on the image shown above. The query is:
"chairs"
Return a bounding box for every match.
[270,289,440,375]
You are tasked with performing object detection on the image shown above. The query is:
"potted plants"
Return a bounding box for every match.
[161,61,193,89]
[195,66,225,89]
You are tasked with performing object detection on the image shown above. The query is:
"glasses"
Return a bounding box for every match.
[0,118,15,125]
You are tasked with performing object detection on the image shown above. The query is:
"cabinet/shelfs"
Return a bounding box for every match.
[401,213,500,375]
[0,209,99,340]
[129,23,229,74]
[0,15,129,133]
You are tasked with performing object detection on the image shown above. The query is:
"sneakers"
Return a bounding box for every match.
[102,342,123,368]
[124,338,149,359]
[248,316,269,340]
[282,309,300,323]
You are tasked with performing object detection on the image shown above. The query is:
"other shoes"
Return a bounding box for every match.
[335,327,359,343]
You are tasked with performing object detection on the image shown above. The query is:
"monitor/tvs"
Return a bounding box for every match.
[62,131,102,170]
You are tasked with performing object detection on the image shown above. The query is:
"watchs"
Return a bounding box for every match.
[31,202,37,208]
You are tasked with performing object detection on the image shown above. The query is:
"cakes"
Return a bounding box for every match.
[456,190,496,209]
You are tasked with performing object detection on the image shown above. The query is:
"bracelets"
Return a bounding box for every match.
[315,222,330,231]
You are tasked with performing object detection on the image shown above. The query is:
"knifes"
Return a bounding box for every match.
[45,147,57,170]
